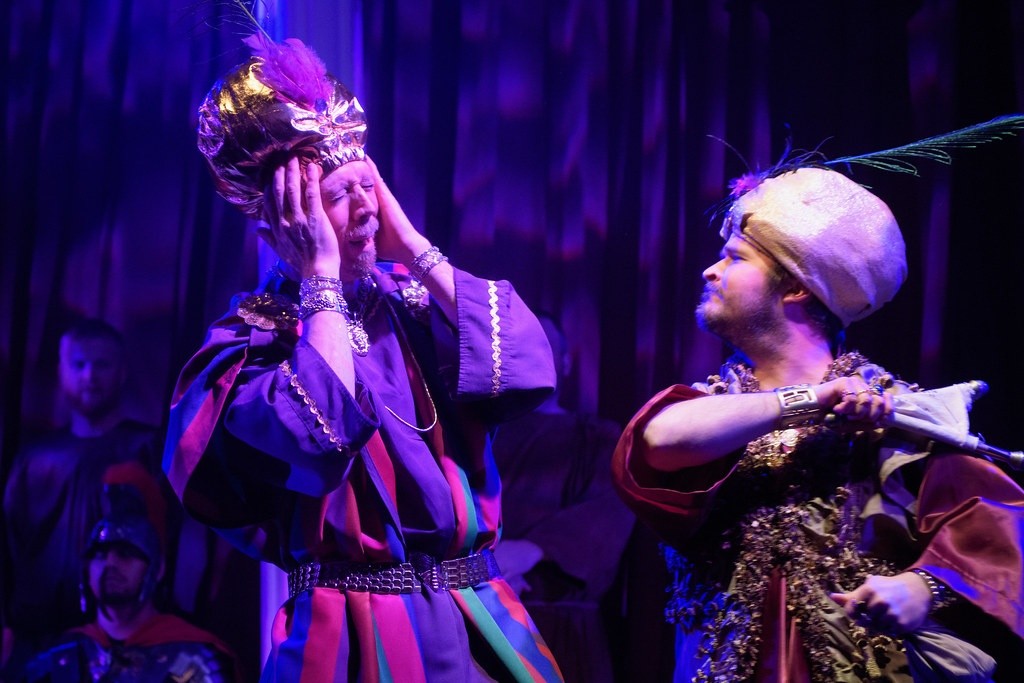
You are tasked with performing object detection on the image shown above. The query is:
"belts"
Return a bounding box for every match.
[286,548,499,600]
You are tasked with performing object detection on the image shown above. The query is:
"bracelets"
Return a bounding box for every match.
[299,275,344,297]
[409,246,449,287]
[773,382,820,431]
[299,291,350,321]
[911,566,944,608]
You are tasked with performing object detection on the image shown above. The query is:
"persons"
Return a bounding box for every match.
[0,316,241,683]
[164,39,558,682]
[612,160,1024,682]
[479,312,640,683]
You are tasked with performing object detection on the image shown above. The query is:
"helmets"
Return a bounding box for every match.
[77,482,164,612]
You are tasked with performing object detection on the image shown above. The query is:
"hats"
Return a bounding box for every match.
[720,166,908,326]
[197,32,367,221]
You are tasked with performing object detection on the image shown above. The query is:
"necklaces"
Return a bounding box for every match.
[345,275,373,355]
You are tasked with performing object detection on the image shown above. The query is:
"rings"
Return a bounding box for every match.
[842,391,856,397]
[870,384,883,396]
[856,389,869,396]
